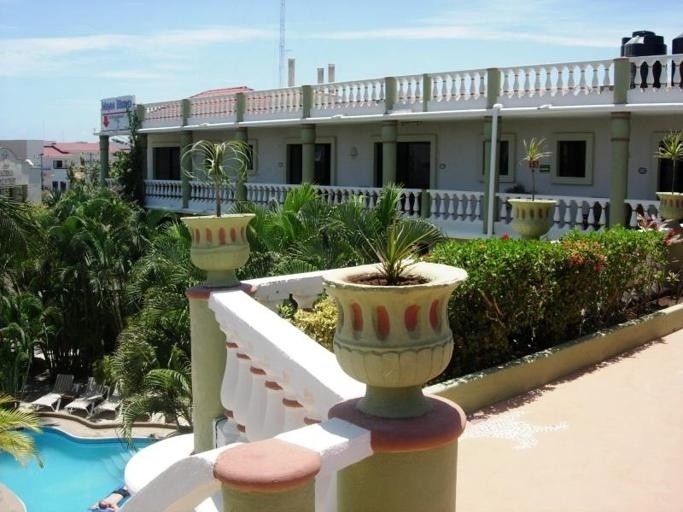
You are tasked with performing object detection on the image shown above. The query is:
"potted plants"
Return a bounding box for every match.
[180,138,257,288]
[321,217,468,419]
[507,136,557,241]
[653,129,683,228]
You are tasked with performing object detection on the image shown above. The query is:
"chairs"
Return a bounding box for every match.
[31,374,157,422]
